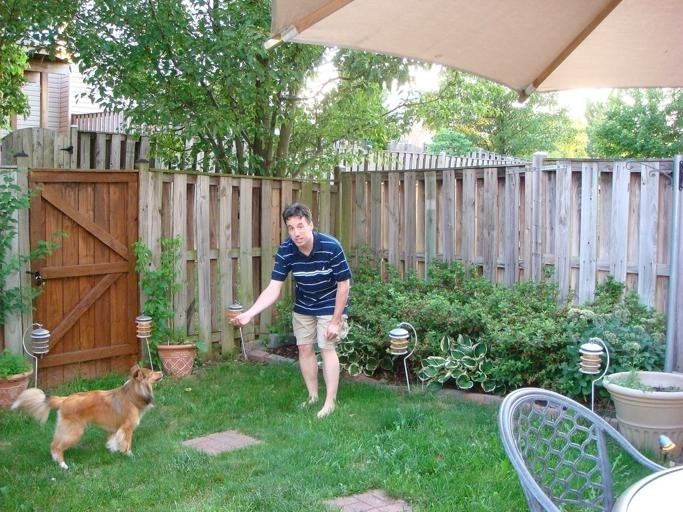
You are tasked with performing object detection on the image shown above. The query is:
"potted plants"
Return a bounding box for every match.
[155,328,196,379]
[0,349,34,414]
[601,342,681,463]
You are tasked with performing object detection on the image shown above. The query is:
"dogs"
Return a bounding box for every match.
[9,362,166,472]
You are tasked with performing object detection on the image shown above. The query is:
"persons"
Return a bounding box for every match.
[228,202,352,419]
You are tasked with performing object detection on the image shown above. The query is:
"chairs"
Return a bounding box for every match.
[499,388,671,512]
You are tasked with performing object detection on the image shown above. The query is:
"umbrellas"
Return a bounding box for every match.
[262,0,682,103]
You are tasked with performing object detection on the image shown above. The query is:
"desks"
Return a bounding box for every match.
[609,466,683,512]
